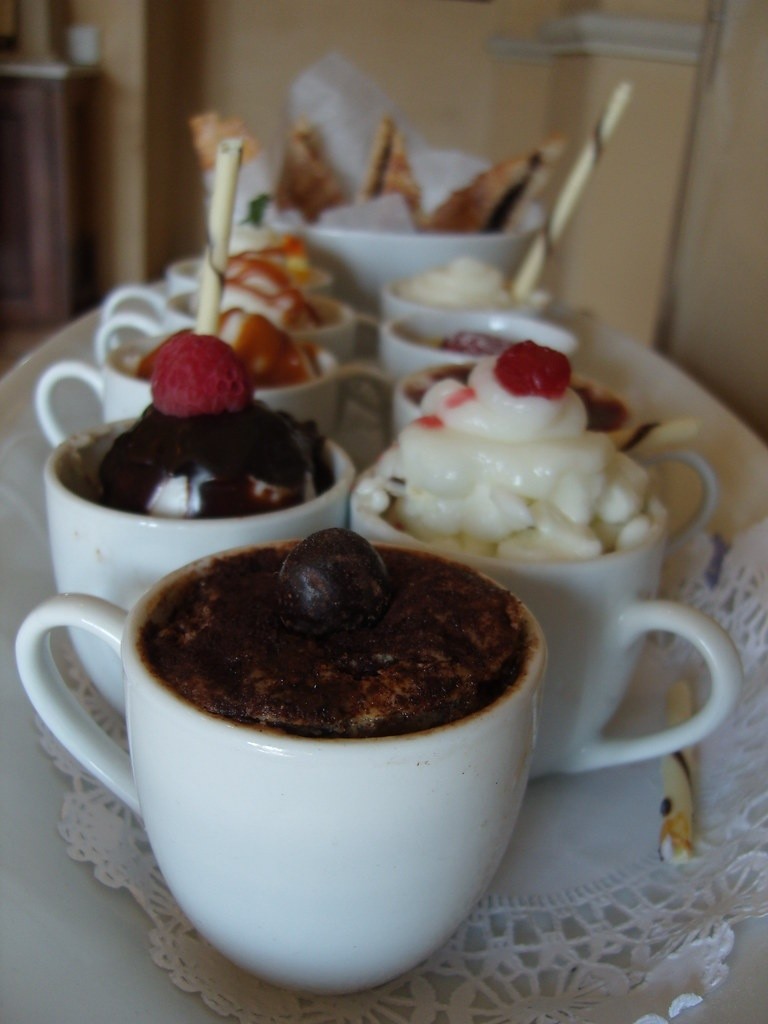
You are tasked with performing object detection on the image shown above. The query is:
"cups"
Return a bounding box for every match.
[41,411,357,721]
[347,458,744,782]
[33,254,717,548]
[14,539,549,996]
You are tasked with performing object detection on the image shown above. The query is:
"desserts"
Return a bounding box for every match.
[120,526,546,741]
[354,255,666,572]
[48,234,359,524]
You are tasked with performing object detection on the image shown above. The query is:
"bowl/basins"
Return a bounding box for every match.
[208,167,542,314]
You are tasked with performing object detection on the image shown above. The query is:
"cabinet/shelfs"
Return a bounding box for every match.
[0,52,99,333]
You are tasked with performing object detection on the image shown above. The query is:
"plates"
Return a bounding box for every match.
[1,220,767,1024]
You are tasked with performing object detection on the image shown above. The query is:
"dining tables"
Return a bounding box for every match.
[1,285,767,1024]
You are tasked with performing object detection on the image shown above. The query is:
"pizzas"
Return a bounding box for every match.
[186,110,571,235]
